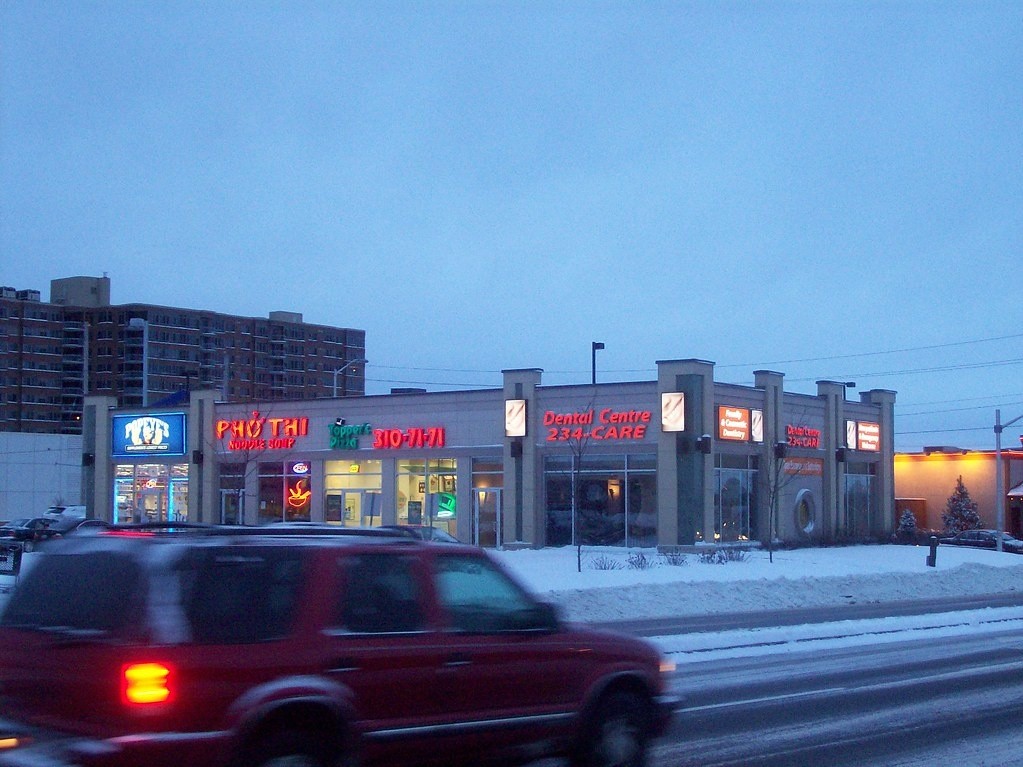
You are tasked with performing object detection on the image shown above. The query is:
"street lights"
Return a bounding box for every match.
[332,358,369,397]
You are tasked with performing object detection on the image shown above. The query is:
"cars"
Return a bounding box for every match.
[0,506,111,555]
[938,529,1023,554]
[0,522,679,767]
